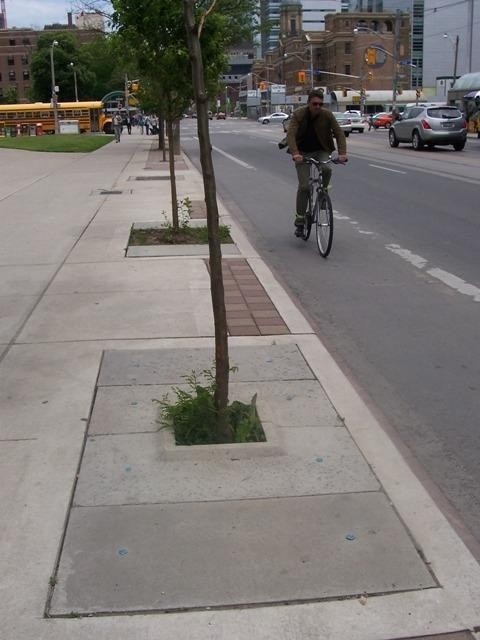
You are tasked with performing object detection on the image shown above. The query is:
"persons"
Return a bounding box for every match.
[368,114,376,130]
[126,113,157,135]
[111,111,124,142]
[279,90,349,237]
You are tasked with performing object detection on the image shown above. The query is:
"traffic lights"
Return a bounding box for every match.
[368,72,373,81]
[416,88,420,97]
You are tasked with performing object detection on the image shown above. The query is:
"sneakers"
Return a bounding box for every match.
[293,213,305,227]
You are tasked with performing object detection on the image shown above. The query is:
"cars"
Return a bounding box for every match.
[329,110,405,138]
[230,110,243,116]
[181,110,226,120]
[257,112,289,125]
[119,113,159,127]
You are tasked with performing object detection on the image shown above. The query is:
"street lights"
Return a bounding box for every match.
[247,67,270,116]
[225,81,241,118]
[443,31,459,105]
[343,89,347,97]
[261,83,264,90]
[300,72,305,82]
[70,63,79,102]
[283,44,314,94]
[49,40,59,135]
[353,8,401,124]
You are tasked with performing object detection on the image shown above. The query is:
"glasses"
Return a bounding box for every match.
[310,101,324,106]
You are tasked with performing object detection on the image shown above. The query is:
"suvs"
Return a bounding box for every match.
[389,103,468,151]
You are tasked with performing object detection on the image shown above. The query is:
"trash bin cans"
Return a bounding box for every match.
[4,123,42,136]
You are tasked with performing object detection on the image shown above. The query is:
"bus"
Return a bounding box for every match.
[102,108,145,118]
[0,101,123,136]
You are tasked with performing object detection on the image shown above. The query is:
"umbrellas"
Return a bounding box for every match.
[463,91,480,102]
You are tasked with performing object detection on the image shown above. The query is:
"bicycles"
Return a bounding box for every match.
[291,154,349,259]
